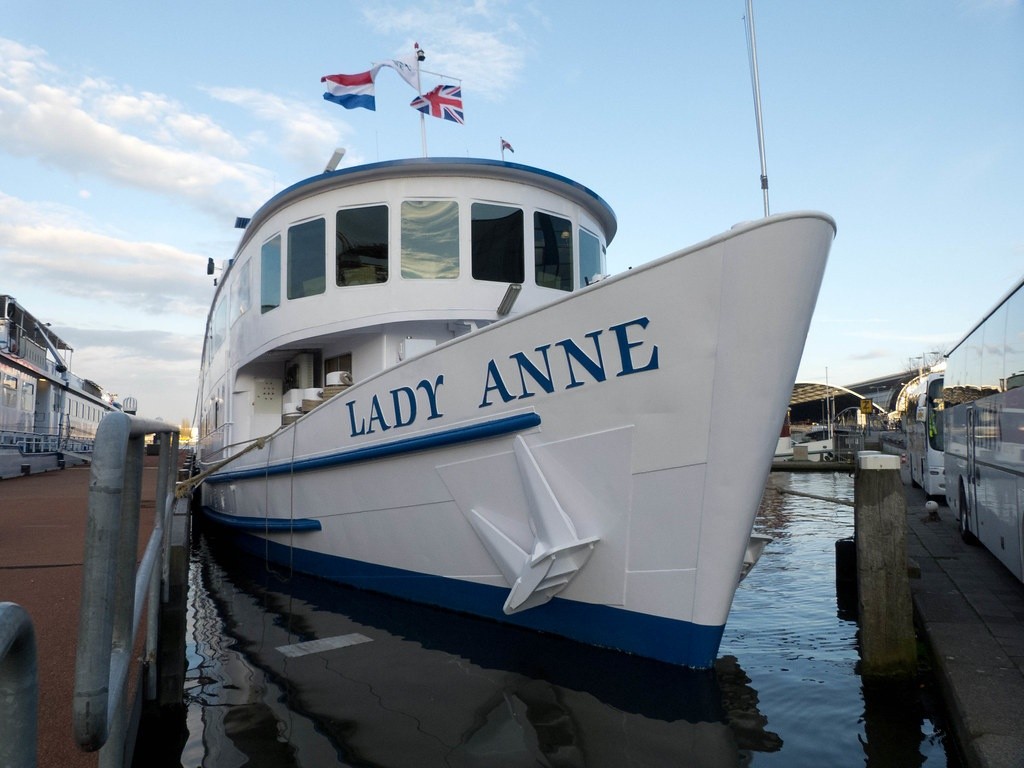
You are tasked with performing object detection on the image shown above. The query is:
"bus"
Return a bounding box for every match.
[943,273,1024,588]
[907,369,946,501]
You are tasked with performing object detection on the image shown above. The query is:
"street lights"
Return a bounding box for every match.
[923,352,940,377]
[909,357,923,380]
[869,386,886,406]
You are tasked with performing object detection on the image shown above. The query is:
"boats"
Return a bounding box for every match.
[0,295,123,481]
[192,40,840,697]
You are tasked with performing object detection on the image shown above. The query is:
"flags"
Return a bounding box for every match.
[410,85,464,124]
[502,140,514,153]
[321,68,376,111]
[370,56,419,92]
